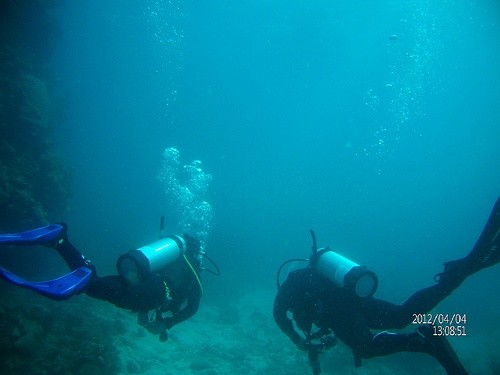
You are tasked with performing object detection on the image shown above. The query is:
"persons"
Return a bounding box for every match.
[39,222,202,335]
[273,263,468,359]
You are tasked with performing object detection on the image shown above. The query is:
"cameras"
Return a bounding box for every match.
[153,317,170,342]
[303,342,325,375]
